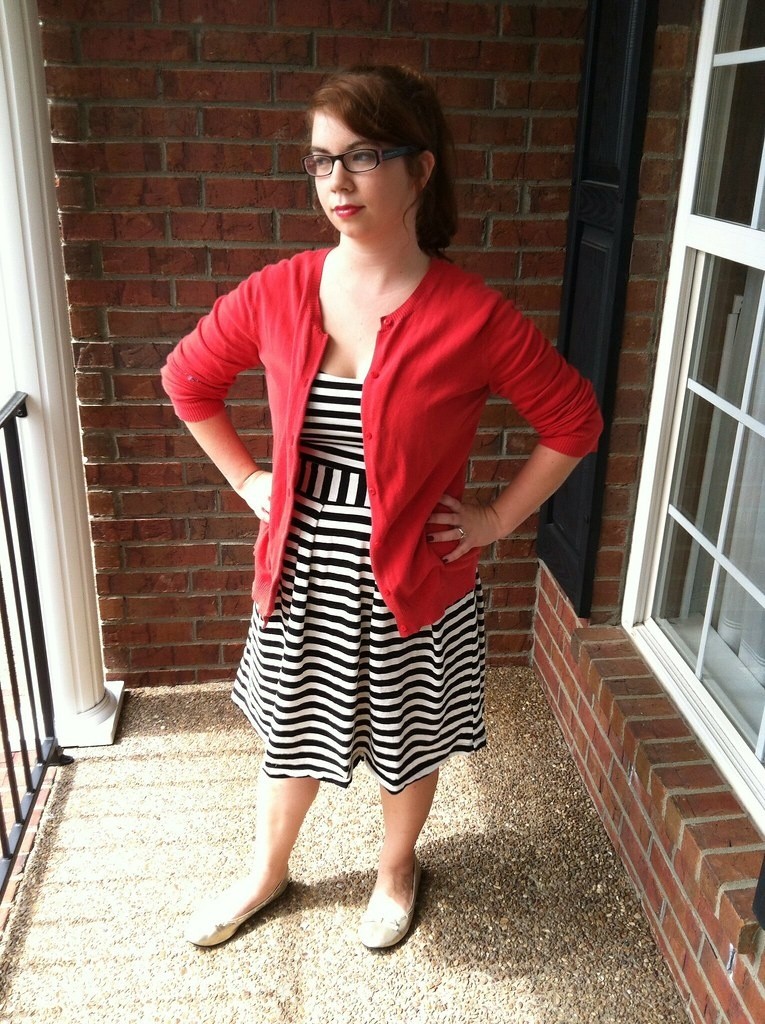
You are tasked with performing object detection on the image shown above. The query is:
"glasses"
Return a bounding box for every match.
[300,147,418,177]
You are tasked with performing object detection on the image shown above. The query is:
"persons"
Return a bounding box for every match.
[161,66,606,953]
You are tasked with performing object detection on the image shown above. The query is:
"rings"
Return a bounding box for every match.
[456,527,468,541]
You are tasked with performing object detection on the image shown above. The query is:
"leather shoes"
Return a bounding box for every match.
[185,864,290,946]
[359,855,421,949]
